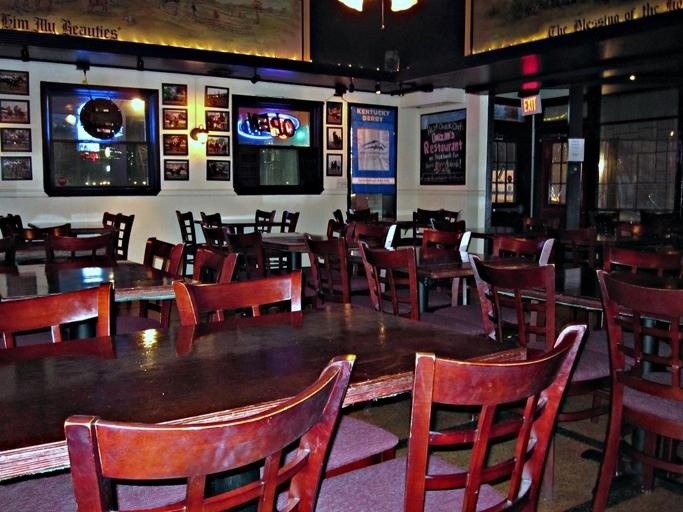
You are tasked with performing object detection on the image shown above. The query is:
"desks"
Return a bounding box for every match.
[396,262,473,314]
[195,219,291,234]
[493,263,682,343]
[1,301,529,511]
[260,231,328,270]
[1,259,203,330]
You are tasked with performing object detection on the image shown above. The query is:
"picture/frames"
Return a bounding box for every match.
[325,101,344,177]
[0,67,31,180]
[160,81,189,182]
[204,84,232,181]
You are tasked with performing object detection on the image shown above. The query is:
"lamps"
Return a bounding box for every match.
[345,76,407,98]
[136,55,146,72]
[20,47,30,62]
[250,67,261,84]
[191,124,209,145]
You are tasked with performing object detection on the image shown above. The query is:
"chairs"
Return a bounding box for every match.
[1,205,682,512]
